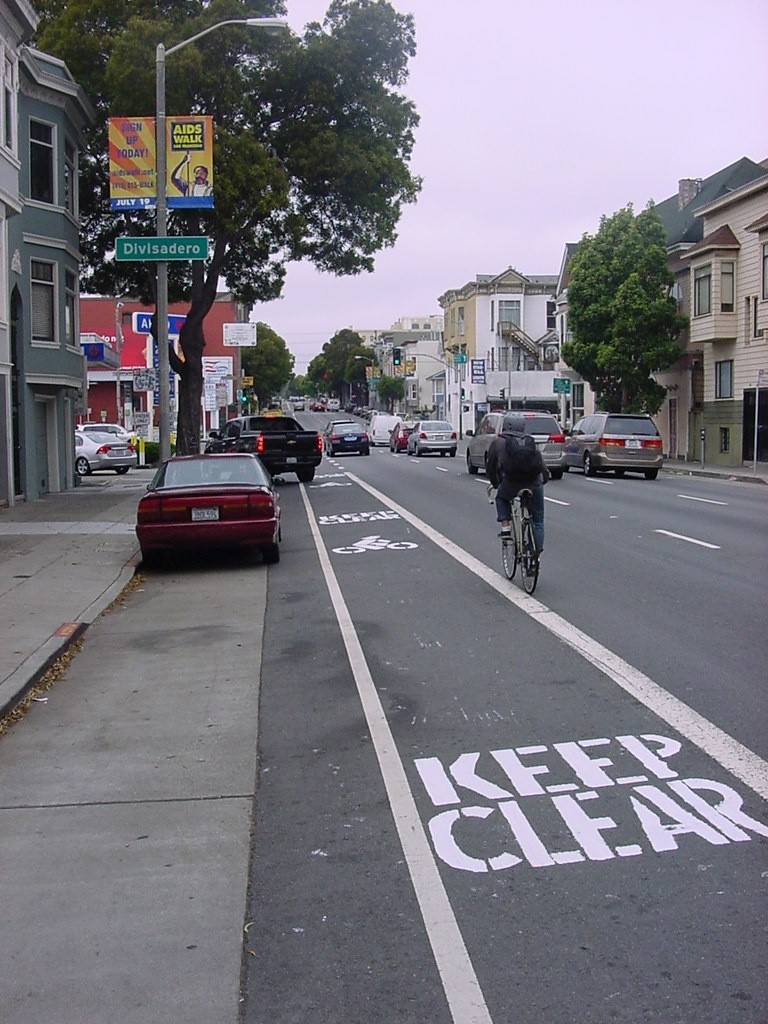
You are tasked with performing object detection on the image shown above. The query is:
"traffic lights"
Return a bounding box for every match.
[393,349,401,366]
[461,389,466,401]
[500,389,504,398]
[243,389,247,402]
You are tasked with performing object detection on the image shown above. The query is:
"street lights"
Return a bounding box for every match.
[157,17,289,442]
[355,355,374,393]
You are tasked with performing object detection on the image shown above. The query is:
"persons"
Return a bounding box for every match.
[486,412,549,577]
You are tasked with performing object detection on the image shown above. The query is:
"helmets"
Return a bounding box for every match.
[502,413,526,432]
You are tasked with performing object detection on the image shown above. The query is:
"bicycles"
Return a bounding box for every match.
[487,469,552,595]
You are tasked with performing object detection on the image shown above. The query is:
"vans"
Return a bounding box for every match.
[430,313,462,439]
[367,416,402,446]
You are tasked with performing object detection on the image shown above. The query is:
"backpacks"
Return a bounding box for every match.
[502,435,542,488]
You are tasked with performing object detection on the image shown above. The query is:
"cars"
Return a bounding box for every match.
[387,421,458,457]
[290,394,390,420]
[325,423,372,457]
[75,423,138,434]
[268,396,283,412]
[136,453,282,568]
[75,430,138,476]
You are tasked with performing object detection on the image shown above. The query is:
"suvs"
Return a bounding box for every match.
[467,410,567,479]
[322,419,355,450]
[566,411,667,480]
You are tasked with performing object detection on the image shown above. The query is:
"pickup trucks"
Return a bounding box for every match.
[204,415,323,483]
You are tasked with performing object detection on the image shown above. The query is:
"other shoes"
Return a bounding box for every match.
[531,556,540,573]
[498,525,513,540]
